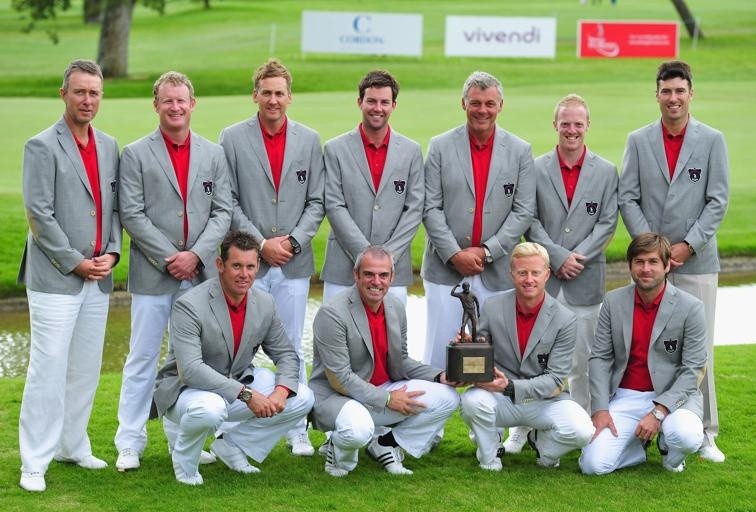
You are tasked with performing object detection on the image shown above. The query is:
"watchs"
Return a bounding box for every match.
[242,386,252,401]
[684,240,694,253]
[288,236,301,253]
[483,245,492,264]
[651,408,666,420]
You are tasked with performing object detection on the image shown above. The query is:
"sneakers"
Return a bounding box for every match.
[116,448,140,472]
[656,432,687,473]
[287,433,314,456]
[55,456,108,469]
[164,442,216,486]
[697,432,725,462]
[468,425,560,472]
[366,435,414,474]
[210,436,260,474]
[19,470,46,492]
[319,435,348,477]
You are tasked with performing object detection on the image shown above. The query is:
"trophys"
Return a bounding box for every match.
[446,281,494,381]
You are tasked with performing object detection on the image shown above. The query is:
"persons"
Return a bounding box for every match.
[451,243,592,470]
[218,59,327,456]
[18,59,119,492]
[619,61,729,463]
[580,233,706,475]
[420,70,538,447]
[114,72,232,468]
[309,246,478,476]
[504,95,617,451]
[154,231,317,485]
[320,70,425,453]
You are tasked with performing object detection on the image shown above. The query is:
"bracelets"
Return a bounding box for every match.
[387,393,391,406]
